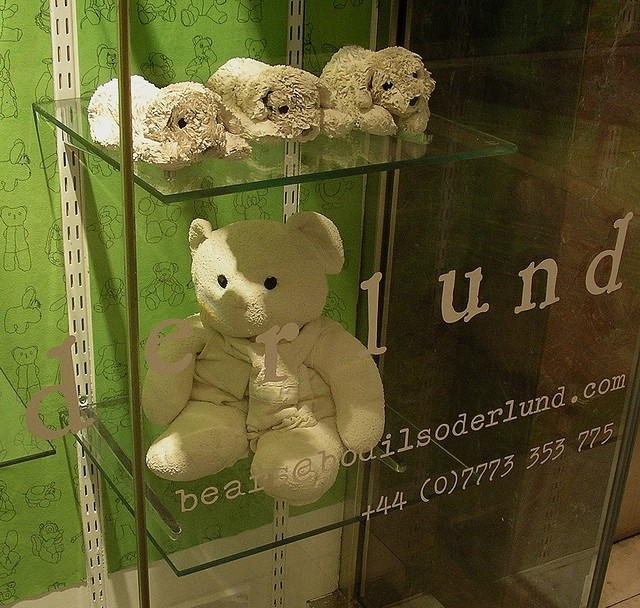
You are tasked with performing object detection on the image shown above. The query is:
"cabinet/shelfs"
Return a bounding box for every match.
[33,1,639,608]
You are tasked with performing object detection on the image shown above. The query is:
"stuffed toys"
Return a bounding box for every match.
[140,211,386,506]
[319,46,437,146]
[87,76,253,170]
[205,58,332,146]
